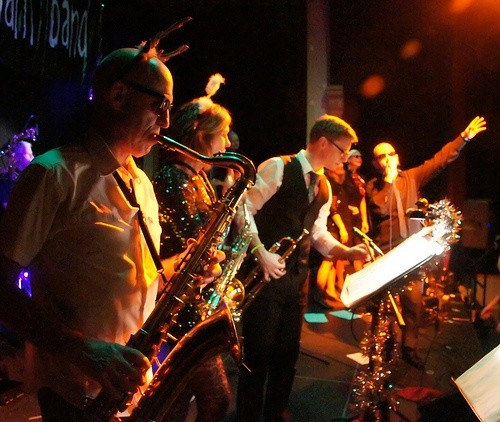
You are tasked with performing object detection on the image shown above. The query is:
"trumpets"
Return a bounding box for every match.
[232,228,310,324]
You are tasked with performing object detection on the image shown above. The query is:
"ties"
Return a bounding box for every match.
[307,171,320,207]
[392,181,407,238]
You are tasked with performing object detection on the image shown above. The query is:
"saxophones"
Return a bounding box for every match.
[214,189,251,318]
[92,135,259,422]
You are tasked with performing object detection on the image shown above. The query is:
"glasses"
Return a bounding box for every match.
[123,81,174,113]
[325,136,348,157]
[351,155,362,158]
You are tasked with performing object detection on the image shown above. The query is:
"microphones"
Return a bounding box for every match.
[350,226,384,257]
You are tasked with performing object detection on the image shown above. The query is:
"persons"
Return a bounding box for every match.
[0,48,226,422]
[232,114,369,422]
[312,116,486,310]
[150,98,231,422]
[1,132,64,387]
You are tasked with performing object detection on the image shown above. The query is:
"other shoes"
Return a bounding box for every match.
[403,348,423,367]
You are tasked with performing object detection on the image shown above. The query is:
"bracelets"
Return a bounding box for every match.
[251,244,264,254]
[460,132,470,143]
